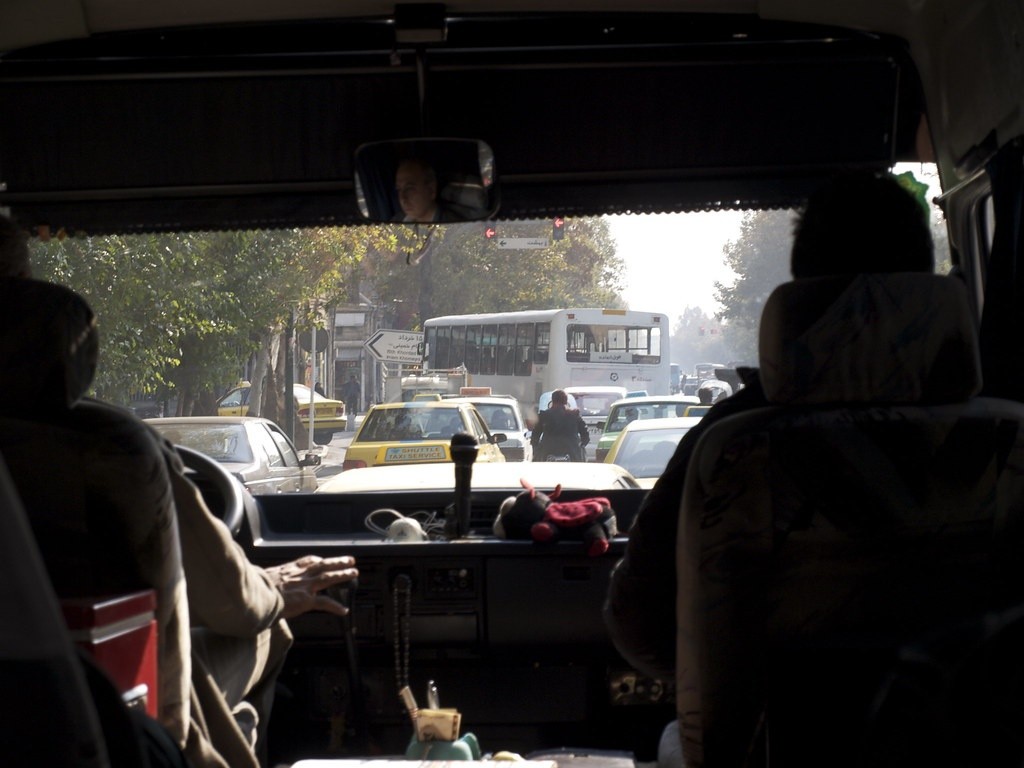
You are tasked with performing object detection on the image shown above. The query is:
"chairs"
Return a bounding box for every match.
[678,269,1024,768]
[0,280,190,747]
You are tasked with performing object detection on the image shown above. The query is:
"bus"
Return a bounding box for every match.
[416,307,671,433]
[670,363,681,394]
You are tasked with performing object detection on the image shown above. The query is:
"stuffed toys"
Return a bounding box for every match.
[493,486,617,555]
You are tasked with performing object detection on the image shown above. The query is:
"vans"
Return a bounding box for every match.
[537,385,627,462]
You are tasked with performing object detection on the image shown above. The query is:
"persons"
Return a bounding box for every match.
[343,375,361,418]
[602,170,1016,768]
[315,382,325,397]
[0,276,359,768]
[530,390,590,462]
[698,388,714,406]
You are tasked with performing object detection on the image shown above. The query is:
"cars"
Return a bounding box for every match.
[342,379,536,476]
[593,395,704,462]
[604,417,707,492]
[216,377,349,448]
[684,404,714,420]
[310,460,644,497]
[140,416,321,497]
[682,377,704,396]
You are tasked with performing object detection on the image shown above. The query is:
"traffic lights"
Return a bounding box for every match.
[552,218,565,240]
[484,222,498,238]
